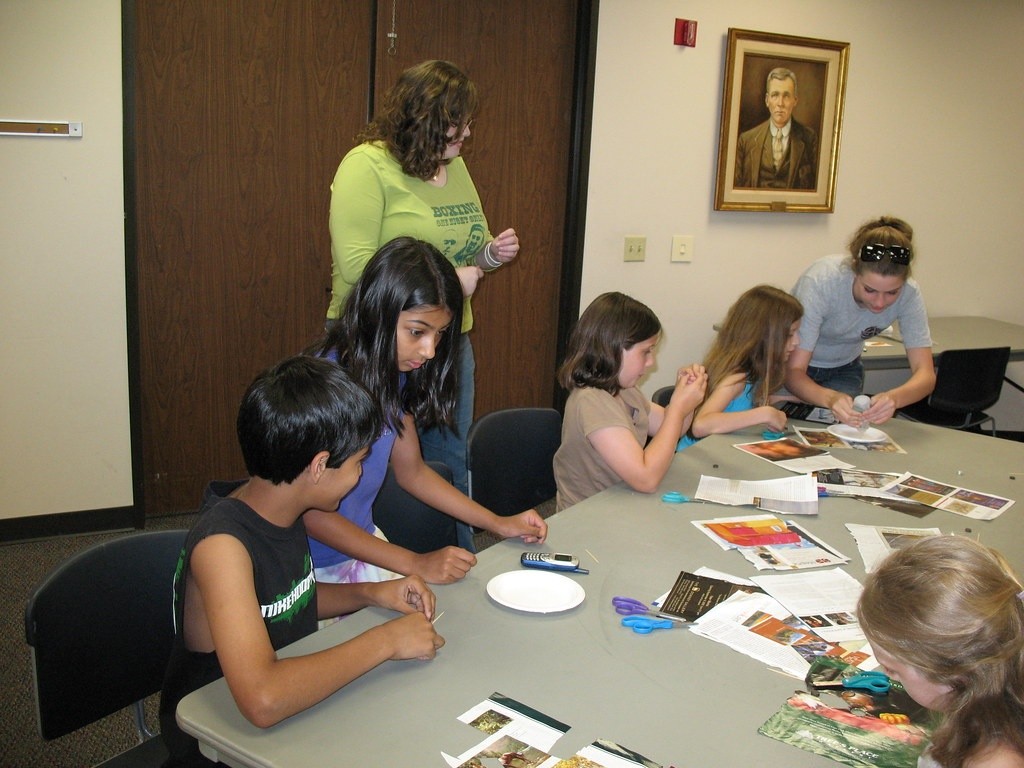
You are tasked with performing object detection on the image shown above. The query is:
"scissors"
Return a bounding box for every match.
[620,616,699,634]
[761,430,796,440]
[611,596,686,622]
[661,491,713,504]
[812,670,891,693]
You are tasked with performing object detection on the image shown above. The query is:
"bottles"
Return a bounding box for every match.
[851,395,871,434]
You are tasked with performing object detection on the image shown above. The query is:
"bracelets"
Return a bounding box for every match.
[484,242,503,267]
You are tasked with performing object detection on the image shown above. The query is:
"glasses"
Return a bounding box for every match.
[860,244,910,266]
[452,118,473,129]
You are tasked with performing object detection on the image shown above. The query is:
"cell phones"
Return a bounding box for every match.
[521,552,589,574]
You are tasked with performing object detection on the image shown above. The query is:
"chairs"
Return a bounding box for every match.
[466,408,561,536]
[370,462,457,555]
[895,347,1011,437]
[26,528,192,768]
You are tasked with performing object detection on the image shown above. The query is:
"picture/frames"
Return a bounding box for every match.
[712,27,850,213]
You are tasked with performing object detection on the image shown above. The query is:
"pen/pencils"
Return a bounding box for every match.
[818,492,856,498]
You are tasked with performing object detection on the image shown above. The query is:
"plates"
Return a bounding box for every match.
[486,569,586,614]
[827,423,888,443]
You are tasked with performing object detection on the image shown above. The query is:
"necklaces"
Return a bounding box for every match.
[433,175,438,181]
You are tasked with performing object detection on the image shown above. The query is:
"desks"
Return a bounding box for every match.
[713,317,1024,371]
[176,400,1024,768]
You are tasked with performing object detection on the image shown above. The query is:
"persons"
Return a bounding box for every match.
[305,236,547,628]
[856,535,1024,768]
[553,291,708,514]
[783,217,936,426]
[327,61,519,553]
[676,284,812,454]
[158,356,445,768]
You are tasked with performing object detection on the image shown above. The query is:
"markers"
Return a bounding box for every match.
[817,487,826,491]
[862,347,867,351]
